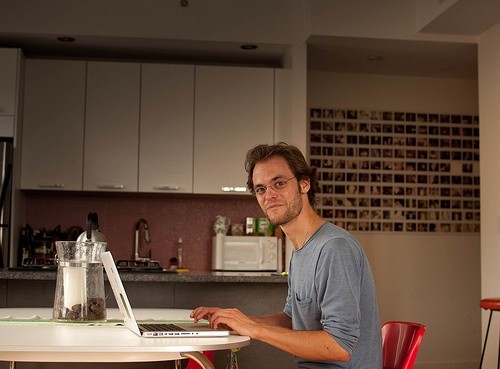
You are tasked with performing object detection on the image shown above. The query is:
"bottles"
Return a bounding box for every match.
[16,224,84,267]
[174,237,186,269]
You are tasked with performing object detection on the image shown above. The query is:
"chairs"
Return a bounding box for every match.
[381,321,426,369]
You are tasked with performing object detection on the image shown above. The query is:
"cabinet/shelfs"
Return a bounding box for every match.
[18,58,88,191]
[193,66,275,196]
[81,61,141,192]
[139,63,195,193]
[0,47,24,270]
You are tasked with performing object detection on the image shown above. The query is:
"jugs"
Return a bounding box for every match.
[213,216,231,236]
[53,241,107,323]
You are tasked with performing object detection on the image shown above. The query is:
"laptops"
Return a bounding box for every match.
[101,251,230,338]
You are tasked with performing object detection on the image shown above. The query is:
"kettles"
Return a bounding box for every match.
[76,212,107,241]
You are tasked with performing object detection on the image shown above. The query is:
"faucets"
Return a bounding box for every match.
[132,218,151,266]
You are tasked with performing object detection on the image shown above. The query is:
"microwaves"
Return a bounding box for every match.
[212,233,282,272]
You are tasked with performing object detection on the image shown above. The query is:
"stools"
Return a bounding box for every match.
[479,298,500,369]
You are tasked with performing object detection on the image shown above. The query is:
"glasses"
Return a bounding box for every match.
[254,176,297,195]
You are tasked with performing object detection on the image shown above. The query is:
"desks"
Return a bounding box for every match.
[0,307,252,369]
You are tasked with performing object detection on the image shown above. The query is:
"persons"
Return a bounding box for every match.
[190,143,382,369]
